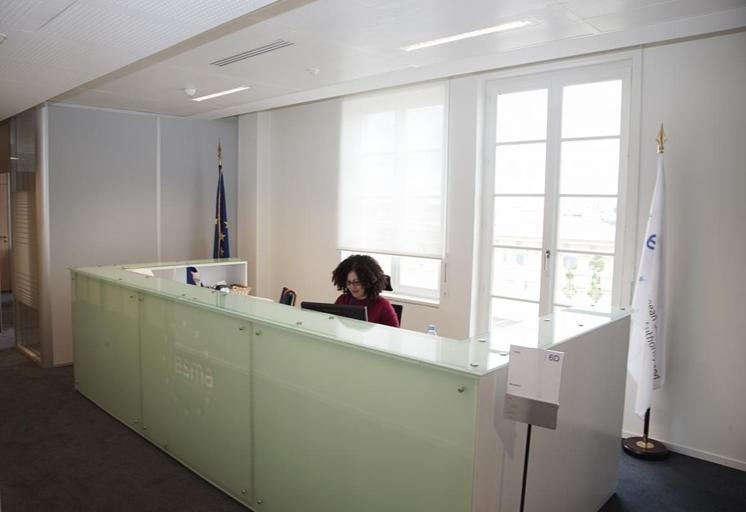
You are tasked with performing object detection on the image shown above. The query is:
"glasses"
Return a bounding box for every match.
[344,279,359,287]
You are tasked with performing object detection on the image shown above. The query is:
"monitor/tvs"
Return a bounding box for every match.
[301,301,369,321]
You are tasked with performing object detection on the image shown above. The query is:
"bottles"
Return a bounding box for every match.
[425,324,437,335]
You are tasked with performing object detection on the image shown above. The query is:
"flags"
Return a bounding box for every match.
[630,151,667,418]
[213,165,230,258]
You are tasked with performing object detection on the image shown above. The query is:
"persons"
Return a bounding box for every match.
[330,253,401,329]
